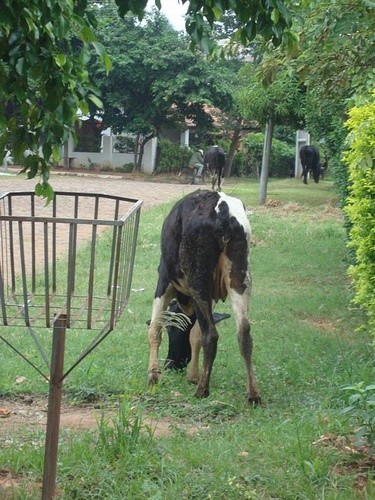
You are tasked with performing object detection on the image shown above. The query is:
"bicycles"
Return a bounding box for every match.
[178,165,205,185]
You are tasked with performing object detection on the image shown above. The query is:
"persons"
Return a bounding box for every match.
[189,148,205,183]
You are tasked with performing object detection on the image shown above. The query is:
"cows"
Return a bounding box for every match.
[298,145,323,185]
[203,144,227,193]
[146,188,262,407]
